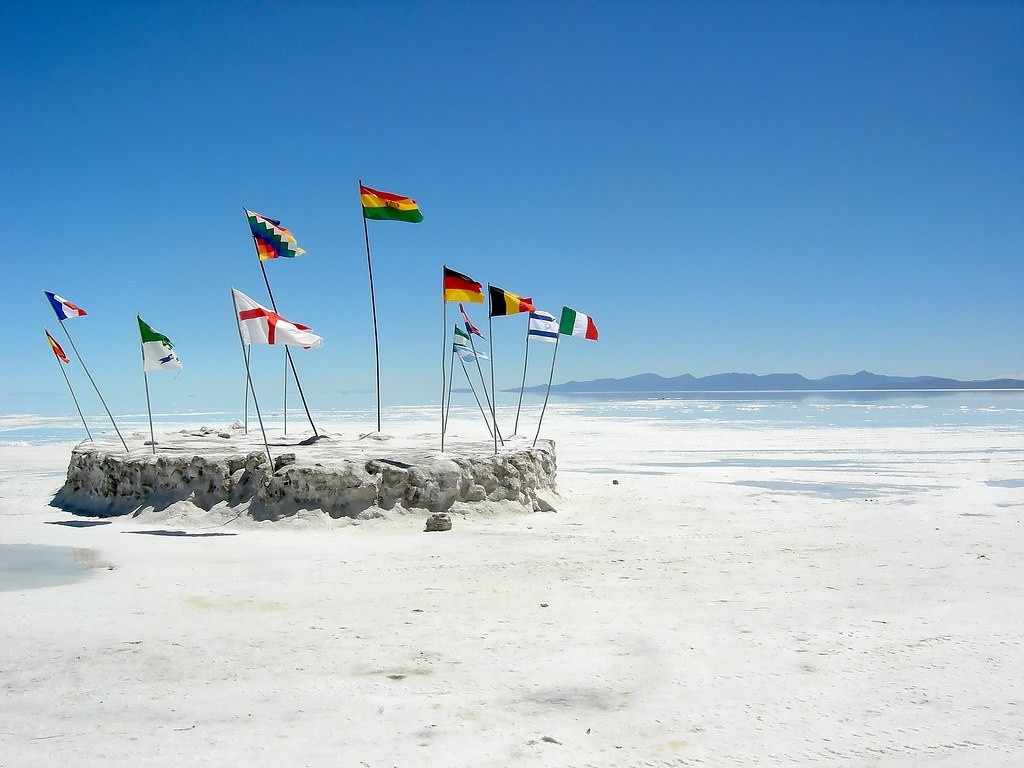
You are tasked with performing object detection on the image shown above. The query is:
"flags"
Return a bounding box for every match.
[246,210,306,260]
[139,320,184,374]
[45,290,87,318]
[361,187,424,223]
[457,346,491,363]
[47,334,69,362]
[560,306,598,341]
[231,289,322,348]
[454,328,469,352]
[460,306,485,339]
[444,268,484,303]
[529,311,559,343]
[489,286,535,316]
